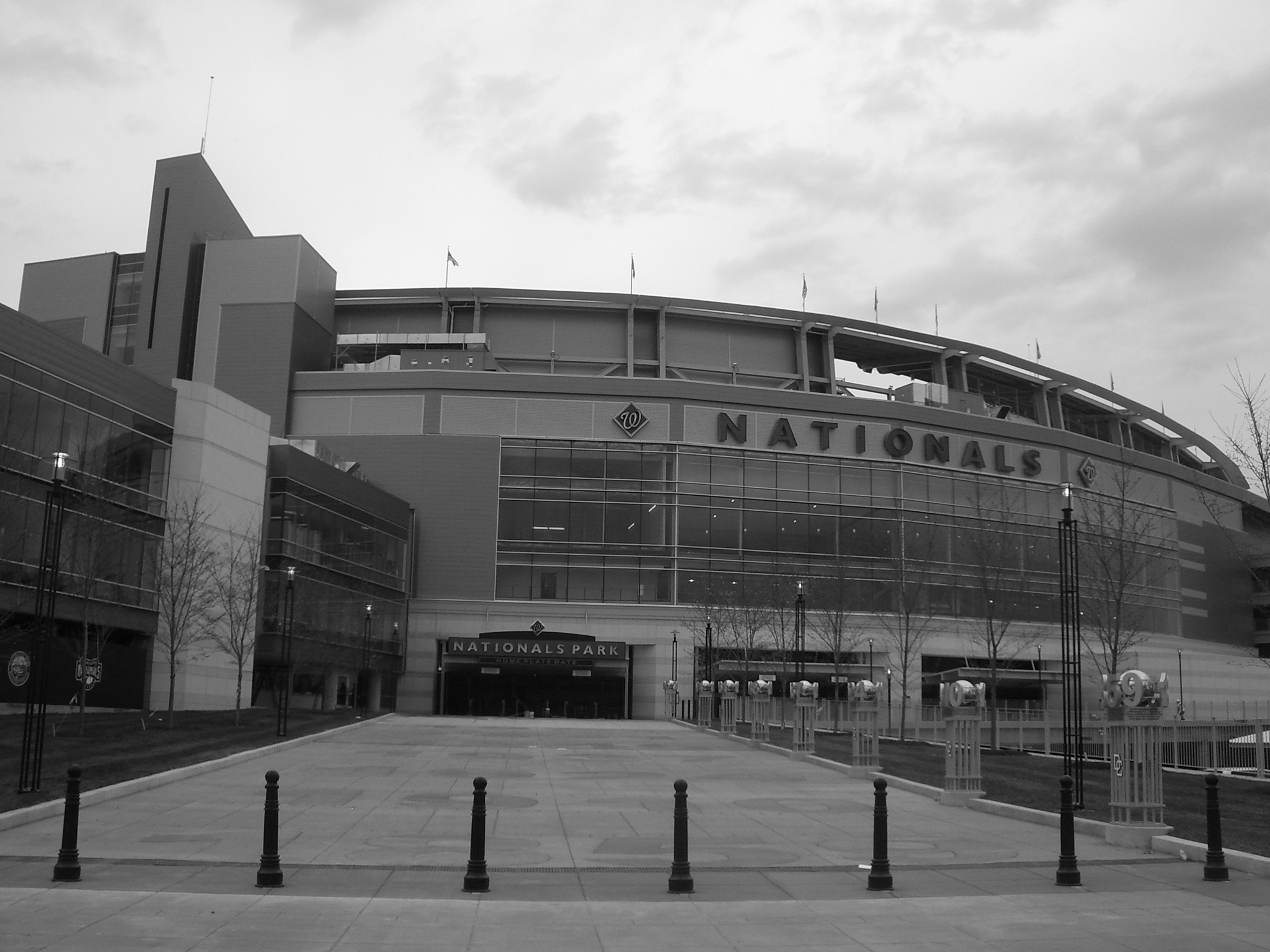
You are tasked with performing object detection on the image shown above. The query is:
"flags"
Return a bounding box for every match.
[802,276,807,298]
[1111,373,1114,391]
[1037,342,1042,360]
[1162,405,1164,415]
[447,249,459,267]
[631,255,635,279]
[874,289,878,311]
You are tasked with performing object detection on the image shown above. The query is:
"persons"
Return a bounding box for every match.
[1175,699,1185,720]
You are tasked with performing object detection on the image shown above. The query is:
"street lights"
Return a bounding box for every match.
[360,604,372,709]
[17,453,70,791]
[796,580,805,682]
[1059,482,1084,813]
[869,638,873,683]
[1037,644,1042,710]
[1179,649,1185,720]
[277,566,296,737]
[705,617,712,682]
[392,621,397,709]
[887,668,892,732]
[670,629,677,718]
